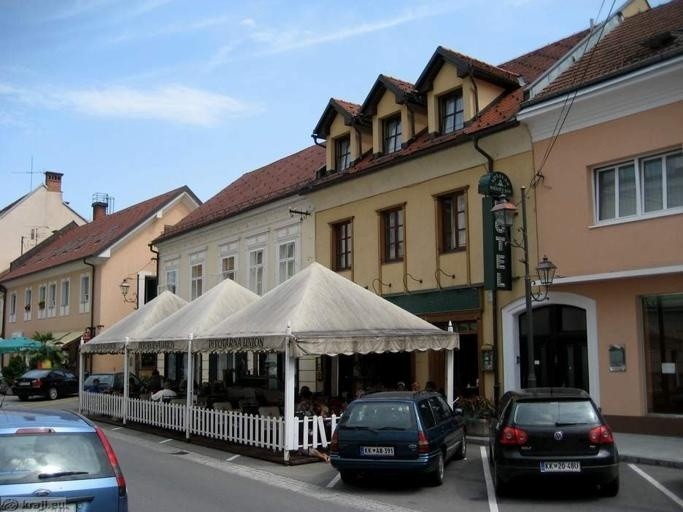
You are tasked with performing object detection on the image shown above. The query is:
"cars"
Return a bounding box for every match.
[83,372,146,396]
[488,387,619,497]
[0,407,128,511]
[12,368,79,401]
[329,389,467,487]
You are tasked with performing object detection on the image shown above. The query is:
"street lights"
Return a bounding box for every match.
[492,185,556,387]
[120,275,139,309]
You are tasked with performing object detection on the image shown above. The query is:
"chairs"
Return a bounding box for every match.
[140,381,285,433]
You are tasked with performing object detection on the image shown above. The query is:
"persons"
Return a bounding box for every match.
[296,381,436,461]
[224,369,264,387]
[86,370,199,403]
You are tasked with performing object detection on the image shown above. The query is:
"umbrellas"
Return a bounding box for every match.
[0,336,43,357]
